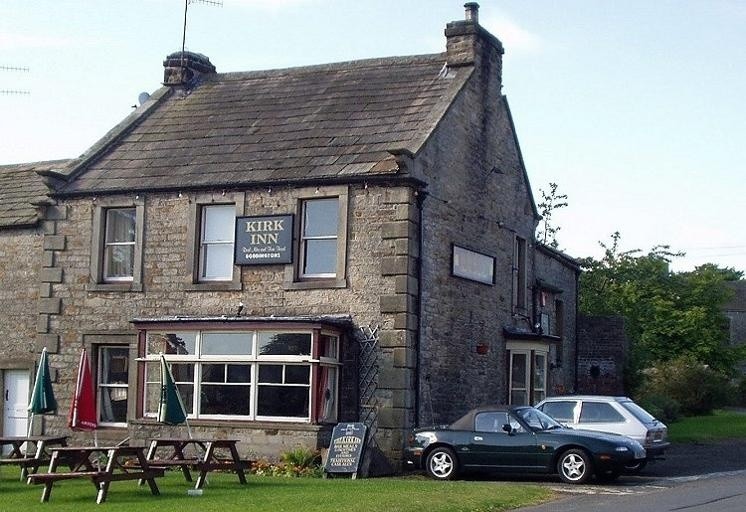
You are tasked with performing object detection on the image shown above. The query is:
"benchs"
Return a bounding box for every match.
[0,436,256,504]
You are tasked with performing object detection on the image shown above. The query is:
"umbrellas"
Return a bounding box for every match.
[19,348,58,482]
[155,351,211,486]
[67,345,101,473]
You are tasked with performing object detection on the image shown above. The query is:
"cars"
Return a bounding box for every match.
[402,404,648,485]
[499,394,673,477]
